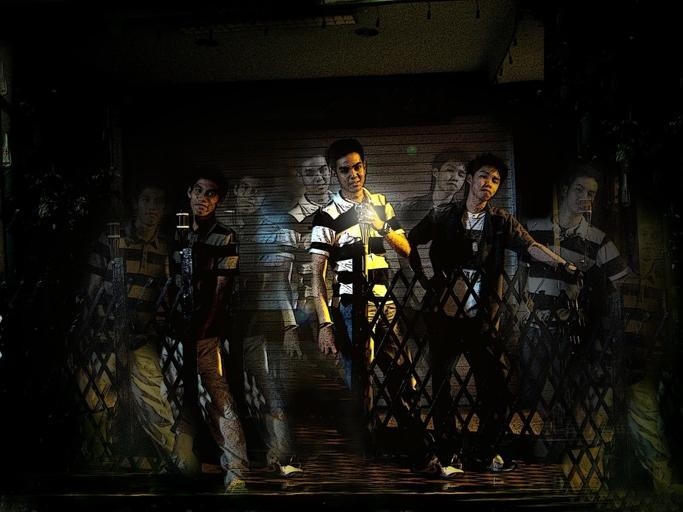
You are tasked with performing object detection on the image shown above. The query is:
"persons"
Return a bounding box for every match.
[580,218,674,492]
[78,175,178,472]
[309,138,435,476]
[217,171,309,480]
[407,156,577,480]
[274,147,340,371]
[390,146,473,382]
[508,173,630,444]
[161,168,251,494]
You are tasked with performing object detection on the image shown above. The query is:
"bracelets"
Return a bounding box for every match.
[283,323,301,332]
[319,320,333,330]
